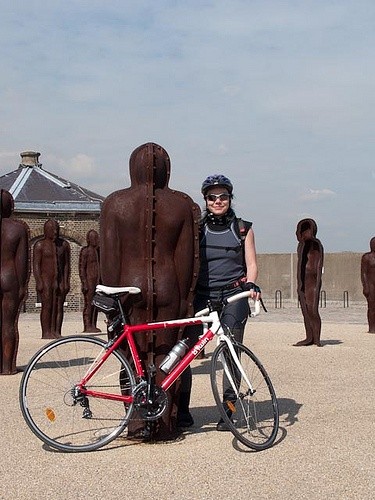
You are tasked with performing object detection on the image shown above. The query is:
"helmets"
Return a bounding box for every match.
[200,174,233,194]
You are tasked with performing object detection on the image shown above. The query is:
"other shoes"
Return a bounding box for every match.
[216,410,232,430]
[176,408,193,427]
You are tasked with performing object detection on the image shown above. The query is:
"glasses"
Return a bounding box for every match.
[205,193,231,201]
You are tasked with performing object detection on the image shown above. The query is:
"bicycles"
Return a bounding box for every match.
[19,285,279,453]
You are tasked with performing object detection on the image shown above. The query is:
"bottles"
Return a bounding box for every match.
[158,337,190,374]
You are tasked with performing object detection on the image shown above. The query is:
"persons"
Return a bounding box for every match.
[360,236,375,333]
[292,218,324,348]
[78,229,104,333]
[99,142,200,442]
[32,218,72,340]
[0,189,31,376]
[175,174,261,434]
